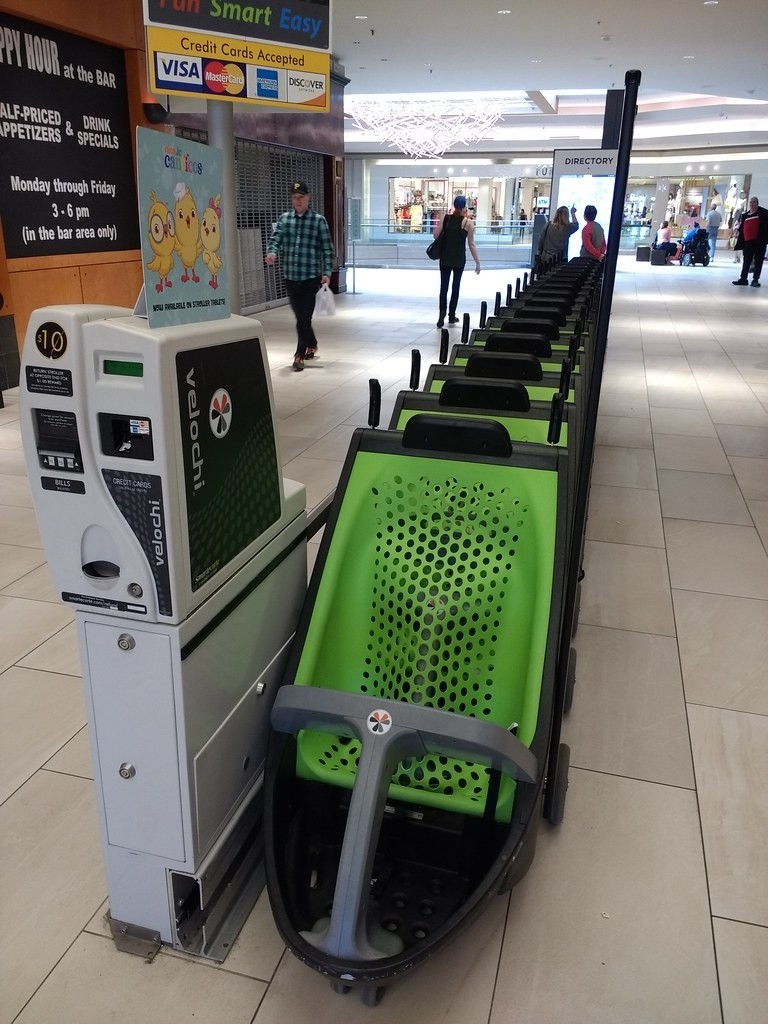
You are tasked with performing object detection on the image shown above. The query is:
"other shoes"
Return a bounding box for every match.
[709,258,714,262]
[751,280,760,287]
[437,316,445,328]
[449,316,459,324]
[666,261,674,266]
[733,260,740,263]
[732,278,749,286]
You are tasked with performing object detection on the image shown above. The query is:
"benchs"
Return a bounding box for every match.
[650,247,666,265]
[636,245,650,261]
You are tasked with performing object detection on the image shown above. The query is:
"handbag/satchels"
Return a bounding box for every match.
[532,254,543,275]
[729,237,737,247]
[315,284,336,316]
[426,215,451,260]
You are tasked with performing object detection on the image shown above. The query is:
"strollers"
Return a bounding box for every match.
[270,257,605,1005]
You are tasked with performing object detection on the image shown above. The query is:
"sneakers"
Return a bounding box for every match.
[304,345,317,359]
[293,352,304,372]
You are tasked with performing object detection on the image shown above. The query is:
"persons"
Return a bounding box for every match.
[728,183,739,228]
[684,222,710,253]
[537,204,579,265]
[266,181,333,372]
[733,190,747,227]
[665,193,675,221]
[706,204,722,262]
[531,208,537,220]
[640,207,647,218]
[652,222,678,266]
[732,197,768,287]
[711,189,722,211]
[580,205,606,261]
[434,196,481,327]
[519,209,528,237]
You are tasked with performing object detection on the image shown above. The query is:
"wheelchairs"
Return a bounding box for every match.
[677,228,711,266]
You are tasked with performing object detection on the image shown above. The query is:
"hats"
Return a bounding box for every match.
[454,196,466,208]
[290,181,308,195]
